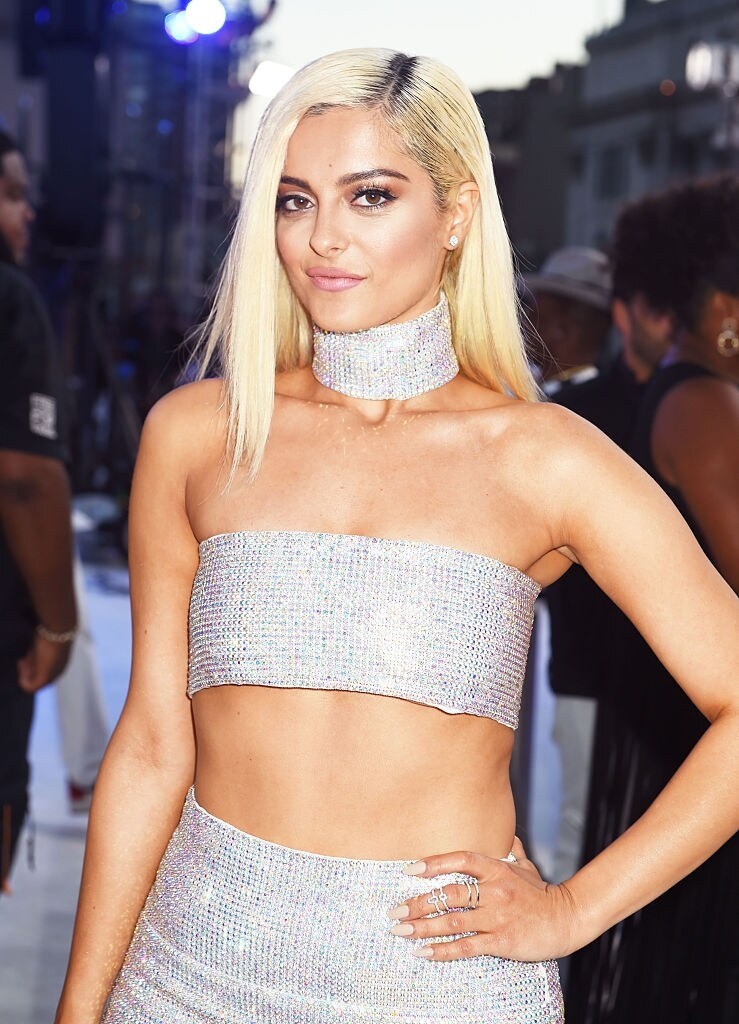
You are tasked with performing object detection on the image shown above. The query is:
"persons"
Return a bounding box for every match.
[0,127,76,889]
[54,45,739,1024]
[521,173,739,1024]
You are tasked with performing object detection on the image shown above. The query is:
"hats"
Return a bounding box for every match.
[517,246,614,314]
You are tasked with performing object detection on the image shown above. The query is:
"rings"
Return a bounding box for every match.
[462,879,481,911]
[427,886,450,913]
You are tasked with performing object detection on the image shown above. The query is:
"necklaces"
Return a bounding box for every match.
[310,292,460,401]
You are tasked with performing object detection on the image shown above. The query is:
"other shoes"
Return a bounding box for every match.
[67,786,98,809]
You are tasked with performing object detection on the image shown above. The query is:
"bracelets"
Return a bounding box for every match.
[38,623,79,644]
